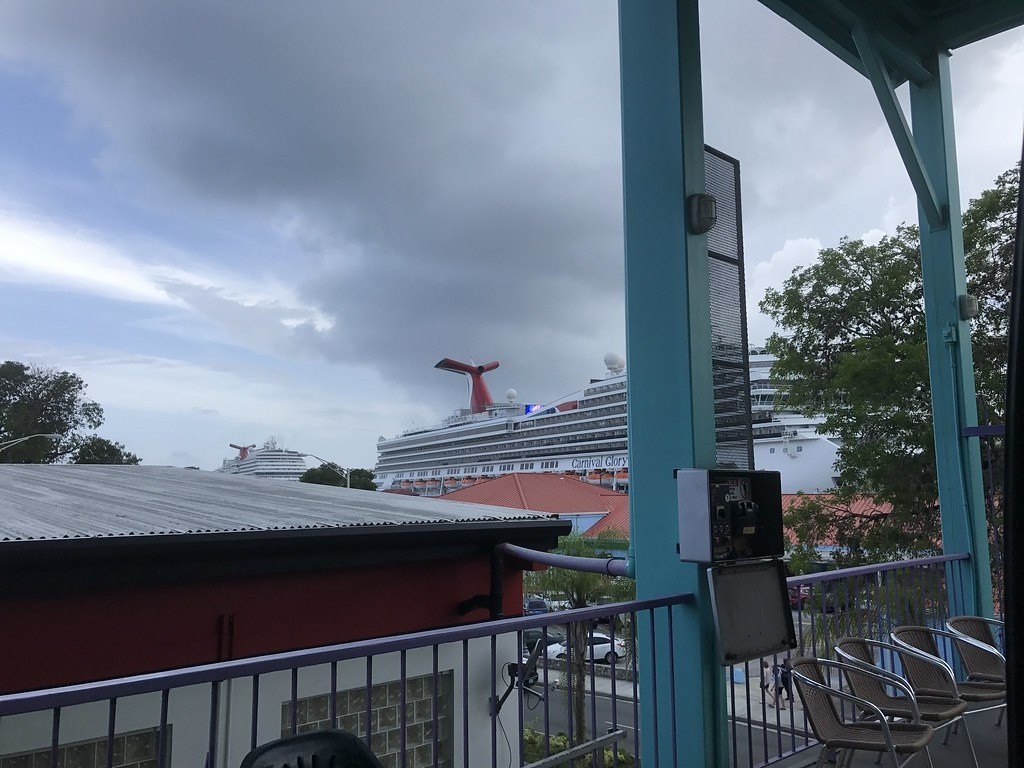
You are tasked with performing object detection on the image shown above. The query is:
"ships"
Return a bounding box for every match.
[371,350,927,501]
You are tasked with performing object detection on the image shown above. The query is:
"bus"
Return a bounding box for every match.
[541,591,614,620]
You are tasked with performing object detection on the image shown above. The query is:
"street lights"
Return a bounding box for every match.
[294,452,352,488]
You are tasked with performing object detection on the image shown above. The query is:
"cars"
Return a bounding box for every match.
[541,632,625,665]
[519,630,564,658]
[524,601,547,619]
[789,580,827,611]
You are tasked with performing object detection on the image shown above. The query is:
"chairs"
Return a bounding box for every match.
[946,616,1006,725]
[787,657,934,768]
[890,625,1006,745]
[833,638,981,768]
